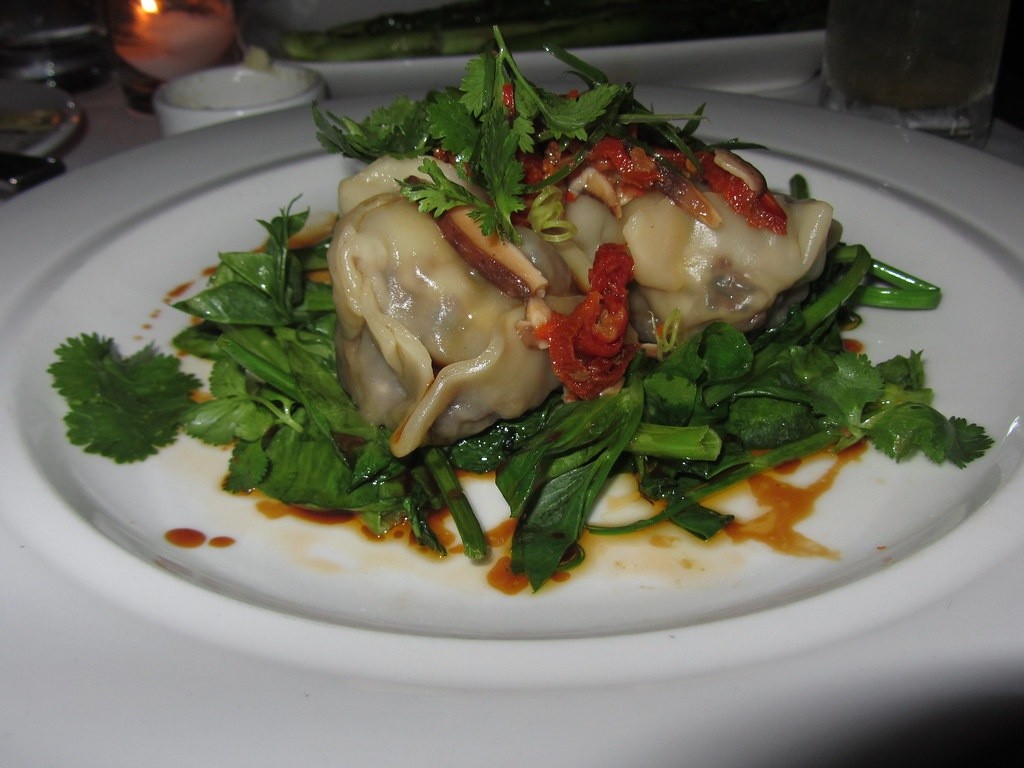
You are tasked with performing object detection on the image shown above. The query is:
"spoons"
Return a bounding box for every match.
[0,151,66,197]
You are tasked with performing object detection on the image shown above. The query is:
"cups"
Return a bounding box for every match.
[105,0,238,119]
[152,60,328,142]
[821,0,1008,148]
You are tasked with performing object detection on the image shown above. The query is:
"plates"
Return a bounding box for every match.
[0,78,82,158]
[223,0,836,93]
[0,80,1024,768]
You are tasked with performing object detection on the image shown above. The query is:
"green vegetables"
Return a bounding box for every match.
[46,194,991,588]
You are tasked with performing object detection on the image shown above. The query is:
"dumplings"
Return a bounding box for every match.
[324,151,842,457]
